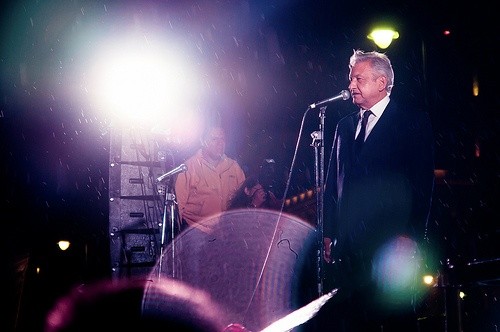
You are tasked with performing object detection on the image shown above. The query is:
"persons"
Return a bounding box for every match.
[168,124,286,230]
[323,51,436,332]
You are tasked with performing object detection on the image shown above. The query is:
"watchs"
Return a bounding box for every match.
[249,203,256,208]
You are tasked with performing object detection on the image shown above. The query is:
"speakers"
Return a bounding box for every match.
[108,126,168,282]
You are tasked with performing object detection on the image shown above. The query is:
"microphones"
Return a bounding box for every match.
[309,90,350,108]
[156,164,188,182]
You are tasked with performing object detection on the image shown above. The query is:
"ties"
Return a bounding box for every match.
[356,110,373,146]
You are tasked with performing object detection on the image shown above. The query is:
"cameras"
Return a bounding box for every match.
[256,158,276,203]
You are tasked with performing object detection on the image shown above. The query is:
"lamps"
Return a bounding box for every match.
[366,0,400,49]
